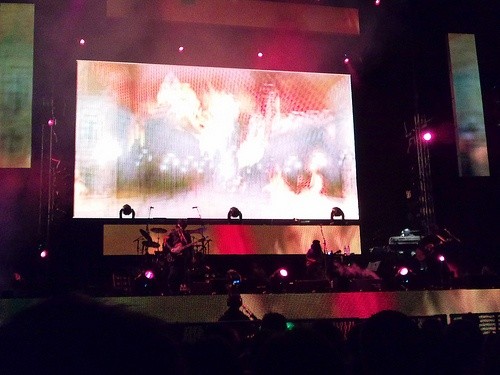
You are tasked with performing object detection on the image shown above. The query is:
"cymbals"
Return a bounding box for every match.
[150,227,167,234]
[139,228,152,241]
[187,228,206,233]
[141,241,160,248]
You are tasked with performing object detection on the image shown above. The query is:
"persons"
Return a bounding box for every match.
[0,292,500,375]
[304,239,323,281]
[159,219,201,295]
[414,242,434,262]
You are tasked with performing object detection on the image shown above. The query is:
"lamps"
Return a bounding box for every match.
[228,207,242,220]
[119,204,136,219]
[331,207,344,221]
[267,267,290,291]
[392,264,408,285]
[132,270,155,292]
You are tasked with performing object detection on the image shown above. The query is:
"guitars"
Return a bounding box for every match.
[169,236,206,255]
[306,250,341,271]
